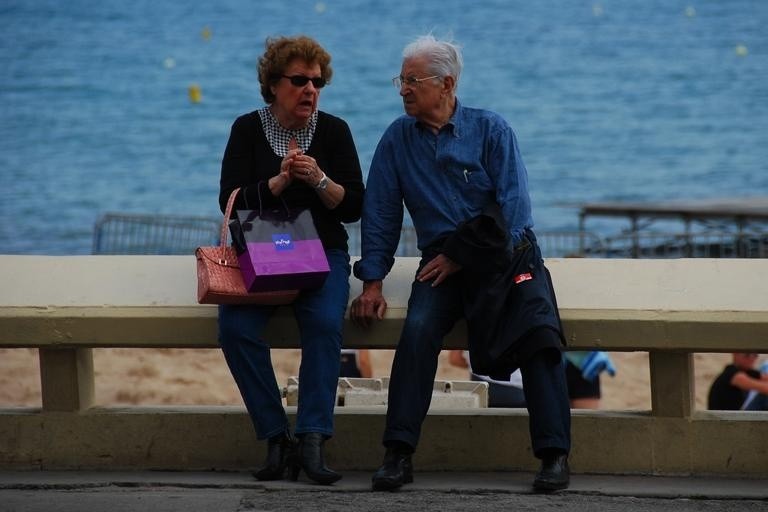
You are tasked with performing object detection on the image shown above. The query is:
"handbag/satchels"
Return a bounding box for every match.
[195,181,331,306]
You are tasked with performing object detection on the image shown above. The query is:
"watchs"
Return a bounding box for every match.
[315,172,328,192]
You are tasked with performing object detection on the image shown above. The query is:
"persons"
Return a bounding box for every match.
[708,353,768,410]
[340,349,372,378]
[351,38,571,491]
[219,36,364,484]
[448,350,617,409]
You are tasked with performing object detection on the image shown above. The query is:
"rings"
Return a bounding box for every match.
[305,170,310,175]
[435,269,438,273]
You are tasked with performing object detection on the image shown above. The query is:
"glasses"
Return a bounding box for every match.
[283,75,326,88]
[392,76,438,89]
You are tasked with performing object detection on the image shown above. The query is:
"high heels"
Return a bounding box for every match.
[251,432,343,485]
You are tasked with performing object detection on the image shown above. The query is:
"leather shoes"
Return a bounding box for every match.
[532,453,570,490]
[371,444,414,490]
